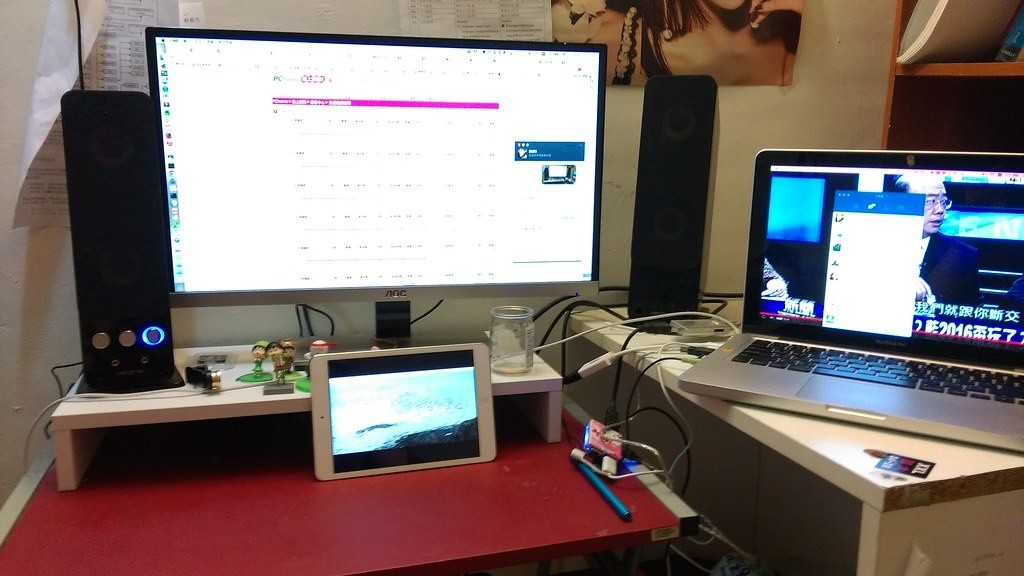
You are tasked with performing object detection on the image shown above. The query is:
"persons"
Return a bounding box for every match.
[894,173,984,306]
[585,0,805,86]
[761,257,787,298]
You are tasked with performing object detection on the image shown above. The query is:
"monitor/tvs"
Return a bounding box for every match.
[144,26,609,339]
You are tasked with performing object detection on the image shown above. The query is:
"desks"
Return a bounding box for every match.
[0,396,699,576]
[567,301,1024,576]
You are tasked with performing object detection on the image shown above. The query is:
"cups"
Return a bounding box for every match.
[489,306,534,374]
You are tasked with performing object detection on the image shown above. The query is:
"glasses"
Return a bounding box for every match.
[924,199,953,209]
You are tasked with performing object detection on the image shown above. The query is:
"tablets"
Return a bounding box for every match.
[310,343,497,480]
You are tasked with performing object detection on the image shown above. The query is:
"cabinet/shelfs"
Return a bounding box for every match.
[882,0,1024,155]
[51,342,562,490]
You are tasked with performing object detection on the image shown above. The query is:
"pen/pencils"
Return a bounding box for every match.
[569,452,632,522]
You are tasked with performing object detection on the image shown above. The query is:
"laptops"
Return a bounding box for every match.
[678,148,1024,453]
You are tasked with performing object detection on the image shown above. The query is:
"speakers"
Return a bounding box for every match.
[625,73,720,327]
[59,89,177,392]
